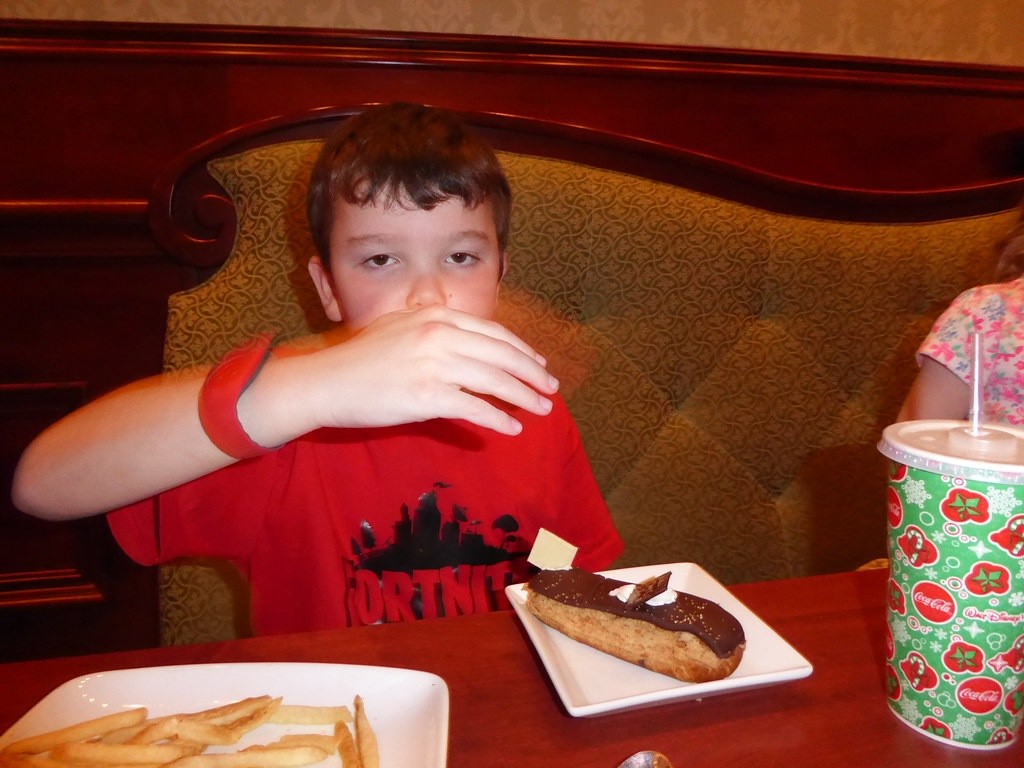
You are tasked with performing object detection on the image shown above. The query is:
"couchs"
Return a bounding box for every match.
[138,100,1024,648]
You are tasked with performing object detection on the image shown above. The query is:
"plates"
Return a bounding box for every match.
[503,562,813,717]
[0,662,448,768]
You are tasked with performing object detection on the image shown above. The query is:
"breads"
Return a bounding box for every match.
[527,528,746,683]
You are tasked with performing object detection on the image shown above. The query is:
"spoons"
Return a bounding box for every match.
[616,752,671,768]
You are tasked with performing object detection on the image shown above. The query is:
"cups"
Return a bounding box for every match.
[876,419,1024,749]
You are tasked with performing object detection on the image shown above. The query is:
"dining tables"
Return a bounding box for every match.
[0,556,1024,768]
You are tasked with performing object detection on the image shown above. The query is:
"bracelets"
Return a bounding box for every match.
[198,326,277,462]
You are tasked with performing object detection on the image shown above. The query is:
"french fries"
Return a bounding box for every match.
[0,693,380,768]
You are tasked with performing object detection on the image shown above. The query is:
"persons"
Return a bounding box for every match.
[891,270,1024,427]
[15,105,620,636]
